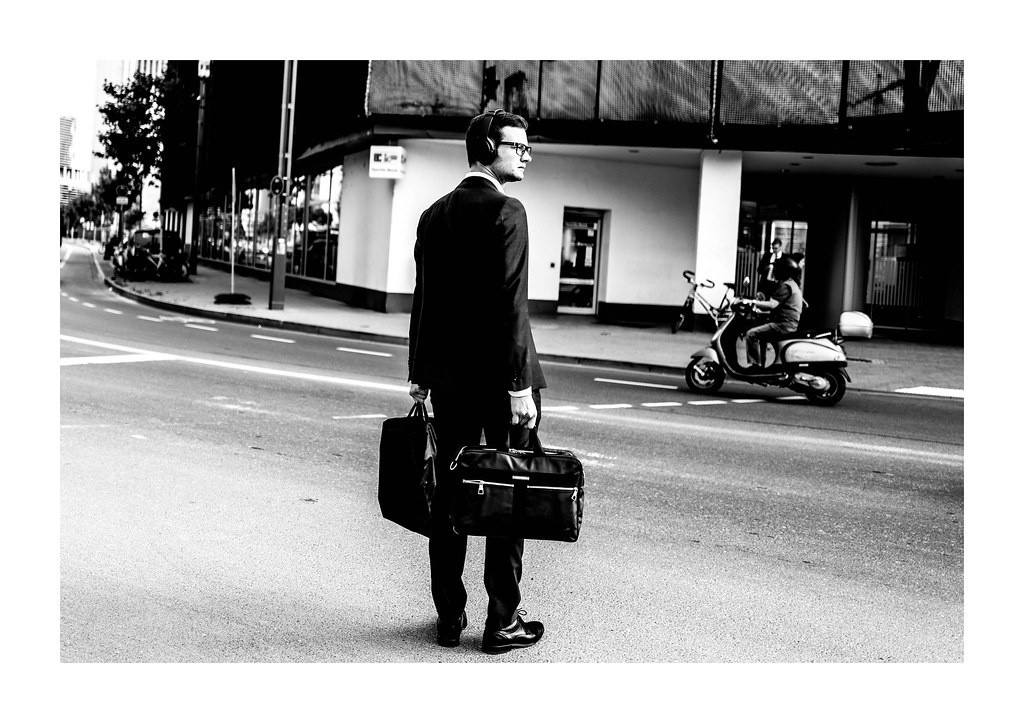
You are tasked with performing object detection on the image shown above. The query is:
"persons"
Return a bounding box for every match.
[755,237,808,340]
[404,109,551,654]
[739,257,804,373]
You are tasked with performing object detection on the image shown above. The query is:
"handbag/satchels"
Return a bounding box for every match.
[446,424,584,542]
[378,402,438,537]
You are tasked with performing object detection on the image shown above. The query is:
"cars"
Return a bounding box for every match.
[110,229,184,282]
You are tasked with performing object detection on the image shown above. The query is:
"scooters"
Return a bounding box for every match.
[685,276,873,406]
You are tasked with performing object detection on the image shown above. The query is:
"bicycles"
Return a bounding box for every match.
[673,270,755,333]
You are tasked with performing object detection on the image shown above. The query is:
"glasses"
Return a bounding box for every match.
[500,142,532,156]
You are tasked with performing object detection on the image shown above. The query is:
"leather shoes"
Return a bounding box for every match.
[437,611,467,647]
[482,608,544,654]
